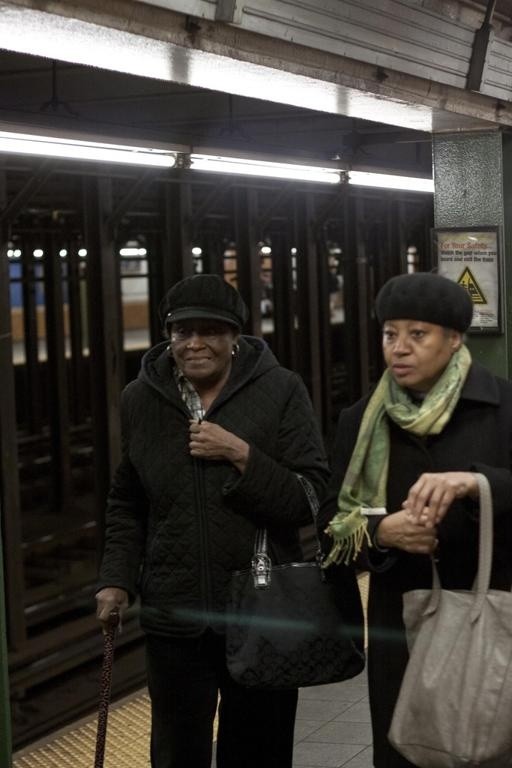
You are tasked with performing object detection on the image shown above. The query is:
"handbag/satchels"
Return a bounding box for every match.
[387,590,512,768]
[217,561,365,691]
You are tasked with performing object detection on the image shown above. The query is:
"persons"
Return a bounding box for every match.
[314,268,512,768]
[93,271,332,768]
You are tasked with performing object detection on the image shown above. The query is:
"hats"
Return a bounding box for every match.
[158,274,249,337]
[375,272,473,335]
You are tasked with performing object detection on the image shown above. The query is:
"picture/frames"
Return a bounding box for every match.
[432,224,505,337]
